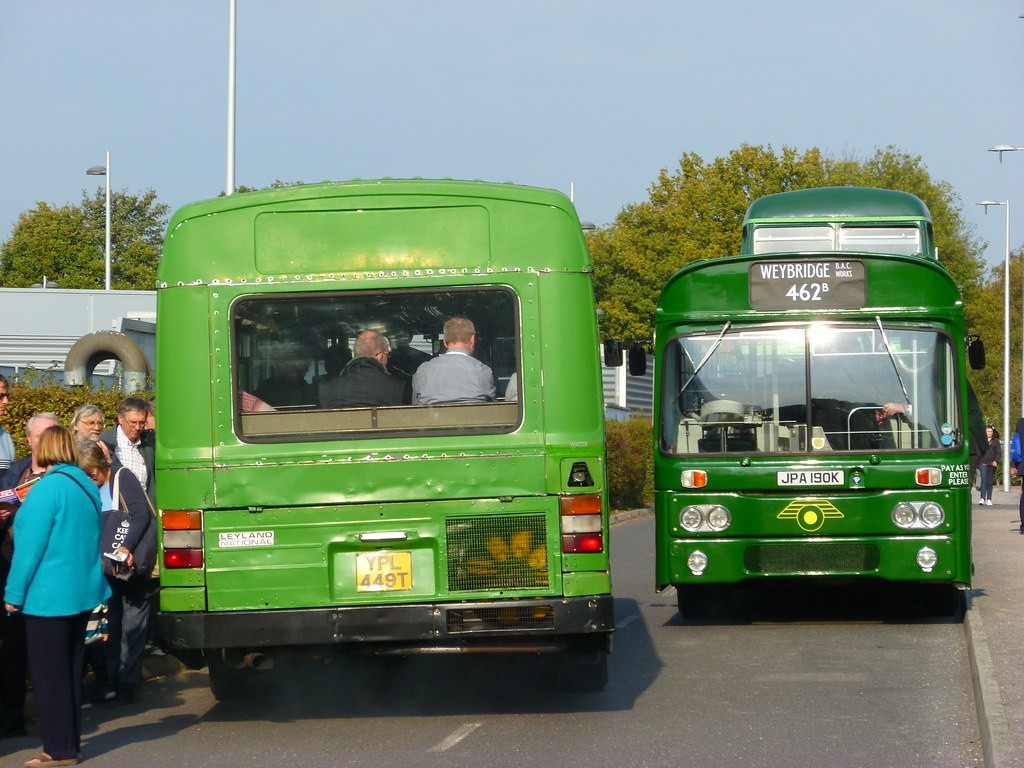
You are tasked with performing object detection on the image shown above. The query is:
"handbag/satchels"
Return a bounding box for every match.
[100,468,158,580]
[976,469,982,491]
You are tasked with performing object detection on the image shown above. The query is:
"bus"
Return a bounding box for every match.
[153,175,615,705]
[739,184,939,266]
[646,249,988,622]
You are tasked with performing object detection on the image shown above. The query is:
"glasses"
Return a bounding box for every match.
[79,420,106,429]
[0,393,10,400]
[123,416,148,426]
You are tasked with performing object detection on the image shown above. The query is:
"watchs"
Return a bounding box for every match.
[902,403,911,417]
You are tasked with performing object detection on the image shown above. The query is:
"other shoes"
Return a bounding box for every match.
[980,499,992,506]
[79,683,116,709]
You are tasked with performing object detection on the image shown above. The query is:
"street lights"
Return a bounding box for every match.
[977,199,1012,494]
[85,148,113,293]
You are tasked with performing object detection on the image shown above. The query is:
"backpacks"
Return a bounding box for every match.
[1011,432,1021,462]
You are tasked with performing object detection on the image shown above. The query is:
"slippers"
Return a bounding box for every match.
[25,752,79,768]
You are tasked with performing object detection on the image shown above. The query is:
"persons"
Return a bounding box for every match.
[975,424,1001,507]
[143,394,156,430]
[0,410,60,738]
[68,404,104,446]
[2,424,114,768]
[95,397,158,541]
[236,288,521,415]
[0,374,16,483]
[873,341,991,492]
[74,436,153,708]
[1010,417,1024,535]
